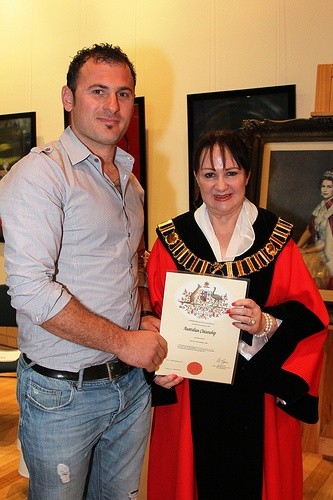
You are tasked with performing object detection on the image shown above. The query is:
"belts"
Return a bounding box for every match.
[22,353,135,382]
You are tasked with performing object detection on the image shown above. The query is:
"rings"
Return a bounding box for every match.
[248,318,256,326]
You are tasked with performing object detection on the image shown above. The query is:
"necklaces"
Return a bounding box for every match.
[98,162,120,183]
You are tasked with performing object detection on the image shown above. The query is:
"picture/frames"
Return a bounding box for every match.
[186,84,296,213]
[1,112,36,246]
[62,95,147,252]
[240,118,333,314]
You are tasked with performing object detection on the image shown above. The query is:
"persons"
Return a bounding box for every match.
[146,129,330,500]
[296,169,333,290]
[0,43,168,500]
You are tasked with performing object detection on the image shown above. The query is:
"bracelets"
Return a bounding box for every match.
[140,311,160,319]
[254,312,272,343]
